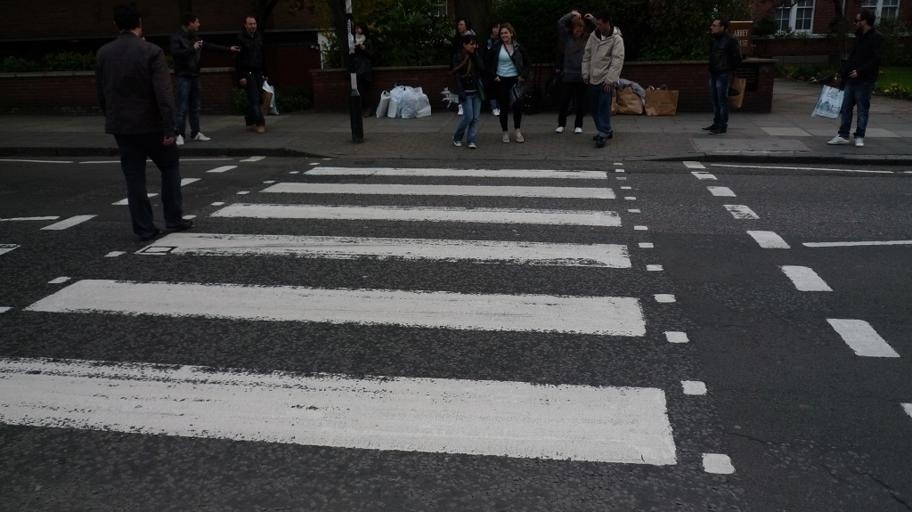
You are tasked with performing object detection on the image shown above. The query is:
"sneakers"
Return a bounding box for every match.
[854,137,865,147]
[453,127,613,148]
[176,134,185,146]
[826,134,851,144]
[457,104,464,116]
[192,132,211,142]
[492,109,501,116]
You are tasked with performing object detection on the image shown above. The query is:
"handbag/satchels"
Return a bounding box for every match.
[811,81,844,120]
[375,85,431,119]
[259,88,272,115]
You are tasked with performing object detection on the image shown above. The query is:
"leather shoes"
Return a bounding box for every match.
[246,124,256,131]
[710,125,728,134]
[167,217,193,230]
[256,125,265,133]
[702,123,715,130]
[139,228,160,241]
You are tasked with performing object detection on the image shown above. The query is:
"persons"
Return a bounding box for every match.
[554,10,599,134]
[826,9,888,148]
[170,12,243,146]
[95,1,193,243]
[701,17,744,136]
[448,18,529,149]
[581,13,625,148]
[235,15,272,134]
[340,20,369,84]
